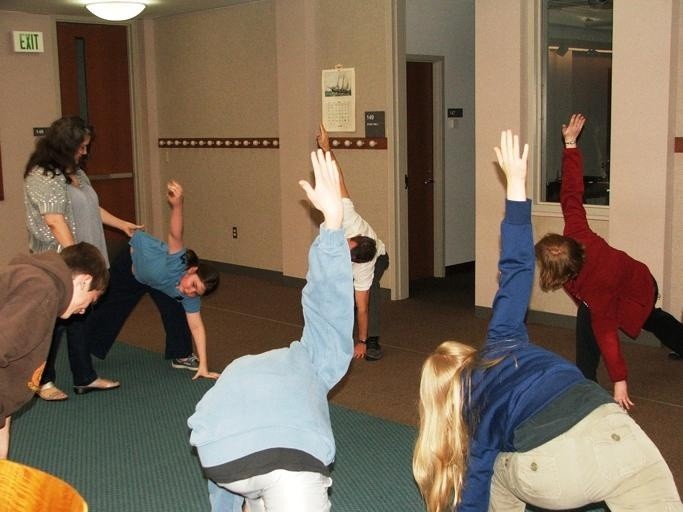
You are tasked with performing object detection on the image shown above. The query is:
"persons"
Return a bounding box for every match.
[82,177,221,381]
[186,147,356,512]
[412,127,682,512]
[24,115,146,402]
[0,241,112,460]
[535,112,683,411]
[316,121,390,361]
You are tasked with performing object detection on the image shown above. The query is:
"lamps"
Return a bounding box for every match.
[84,2,146,21]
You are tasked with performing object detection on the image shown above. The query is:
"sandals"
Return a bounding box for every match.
[74,376,119,394]
[34,382,68,401]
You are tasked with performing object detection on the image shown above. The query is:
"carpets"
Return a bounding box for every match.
[7,329,425,512]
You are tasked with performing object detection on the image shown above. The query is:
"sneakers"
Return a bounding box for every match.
[171,354,200,371]
[364,347,382,360]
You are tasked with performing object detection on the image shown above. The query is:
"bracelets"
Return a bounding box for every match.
[357,339,367,344]
[566,140,576,145]
